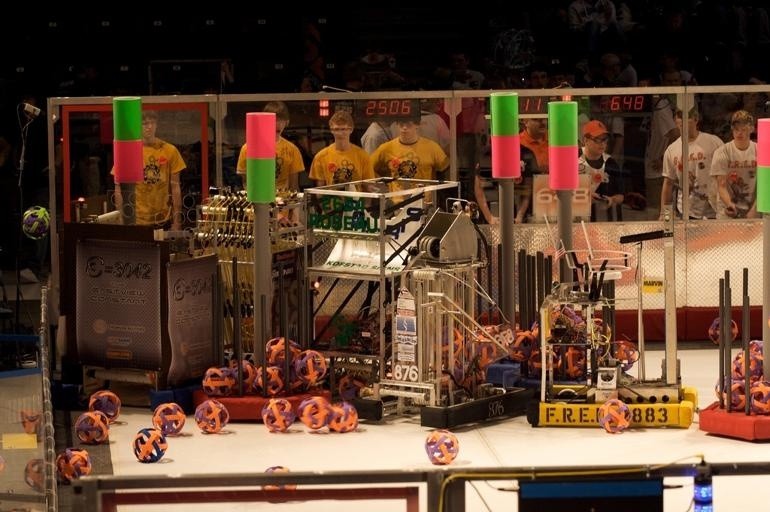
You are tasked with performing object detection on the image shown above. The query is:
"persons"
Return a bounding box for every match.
[577,121,626,221]
[472,142,539,225]
[110,111,187,231]
[306,110,376,212]
[371,116,451,208]
[235,100,306,225]
[642,78,770,220]
[71,2,769,165]
[2,1,106,284]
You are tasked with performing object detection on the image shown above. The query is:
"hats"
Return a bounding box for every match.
[584,120,609,138]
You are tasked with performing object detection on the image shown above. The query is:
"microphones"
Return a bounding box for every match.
[23,103,41,116]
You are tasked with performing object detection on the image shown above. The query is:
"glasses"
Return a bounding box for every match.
[587,137,609,144]
